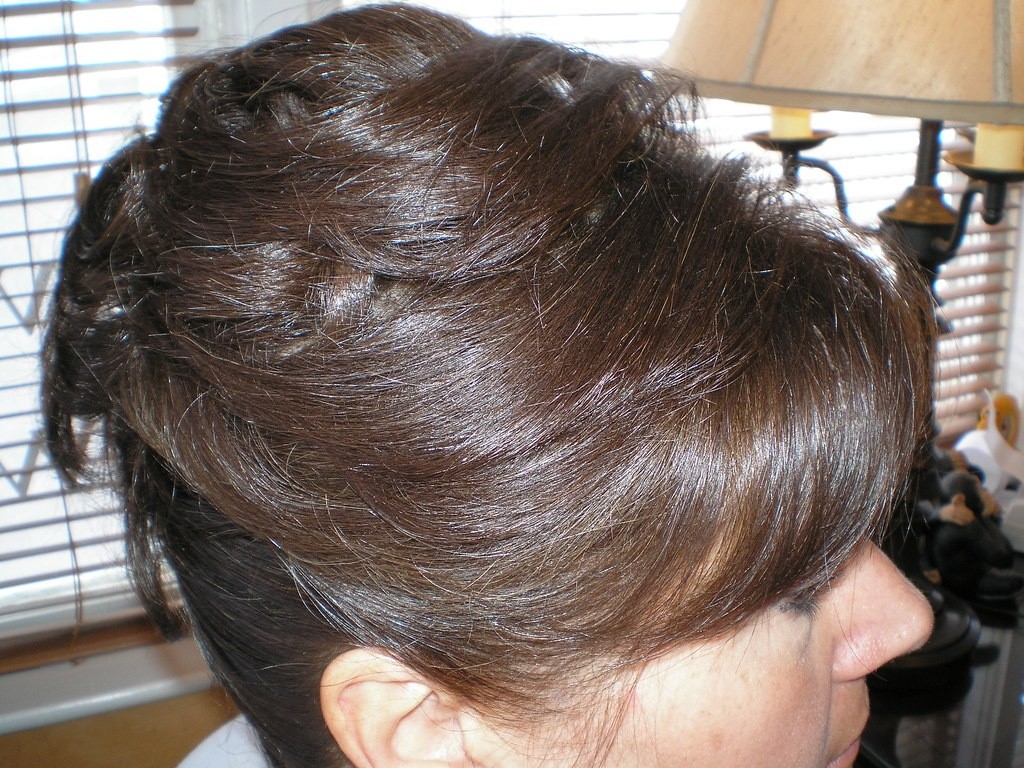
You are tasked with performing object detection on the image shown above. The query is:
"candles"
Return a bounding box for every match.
[767,107,815,139]
[971,120,1024,169]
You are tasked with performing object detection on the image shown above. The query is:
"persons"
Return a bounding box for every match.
[41,3,932,768]
[866,423,1024,672]
